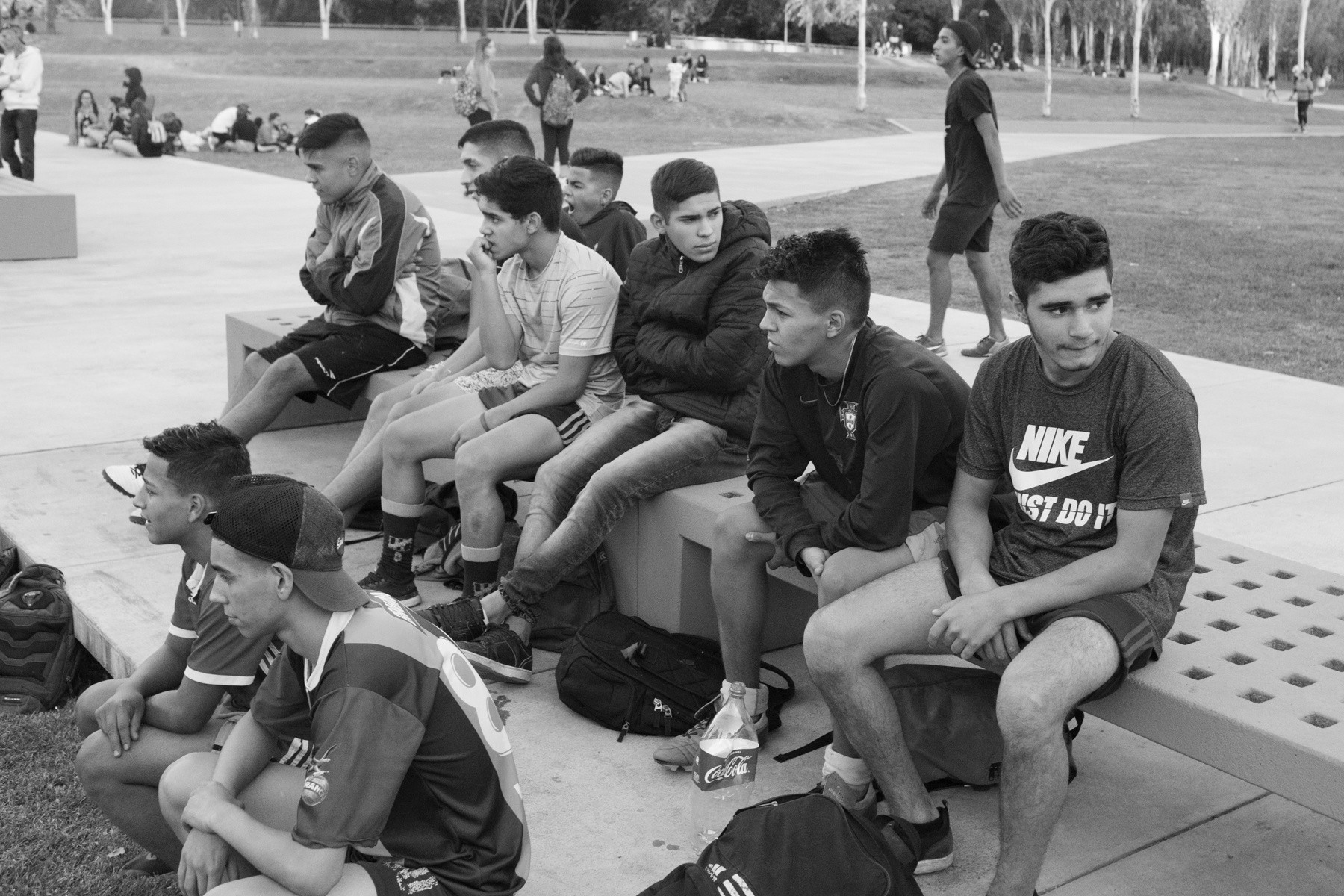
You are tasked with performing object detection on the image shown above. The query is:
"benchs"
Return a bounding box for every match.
[637,460,1344,823]
[0,170,76,260]
[225,306,637,620]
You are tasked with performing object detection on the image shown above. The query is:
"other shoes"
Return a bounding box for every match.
[130,509,147,527]
[101,464,148,498]
[894,799,957,875]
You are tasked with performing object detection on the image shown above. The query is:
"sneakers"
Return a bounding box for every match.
[962,334,1009,360]
[120,852,177,880]
[357,572,422,608]
[914,333,948,359]
[412,596,486,639]
[655,712,771,768]
[807,773,878,822]
[450,619,534,684]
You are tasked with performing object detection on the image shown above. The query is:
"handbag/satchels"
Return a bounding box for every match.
[882,665,1084,788]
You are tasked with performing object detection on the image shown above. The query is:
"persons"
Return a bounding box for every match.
[416,157,773,685]
[654,224,971,819]
[74,421,313,879]
[103,117,441,527]
[465,37,499,125]
[1082,61,1125,77]
[208,103,320,153]
[354,154,622,606]
[1266,60,1332,131]
[0,25,43,181]
[629,28,665,47]
[319,120,587,529]
[573,58,652,97]
[564,147,647,284]
[70,67,166,157]
[990,42,1018,70]
[805,212,1207,896]
[666,51,708,103]
[915,20,1022,357]
[157,474,532,896]
[525,36,590,177]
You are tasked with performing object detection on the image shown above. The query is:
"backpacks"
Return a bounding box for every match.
[635,790,924,896]
[555,611,798,736]
[453,80,477,116]
[138,113,168,144]
[542,68,576,127]
[1,565,82,714]
[502,524,616,649]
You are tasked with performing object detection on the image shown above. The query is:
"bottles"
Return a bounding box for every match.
[688,681,760,858]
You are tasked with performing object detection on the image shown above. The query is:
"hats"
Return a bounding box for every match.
[946,19,980,71]
[204,473,371,612]
